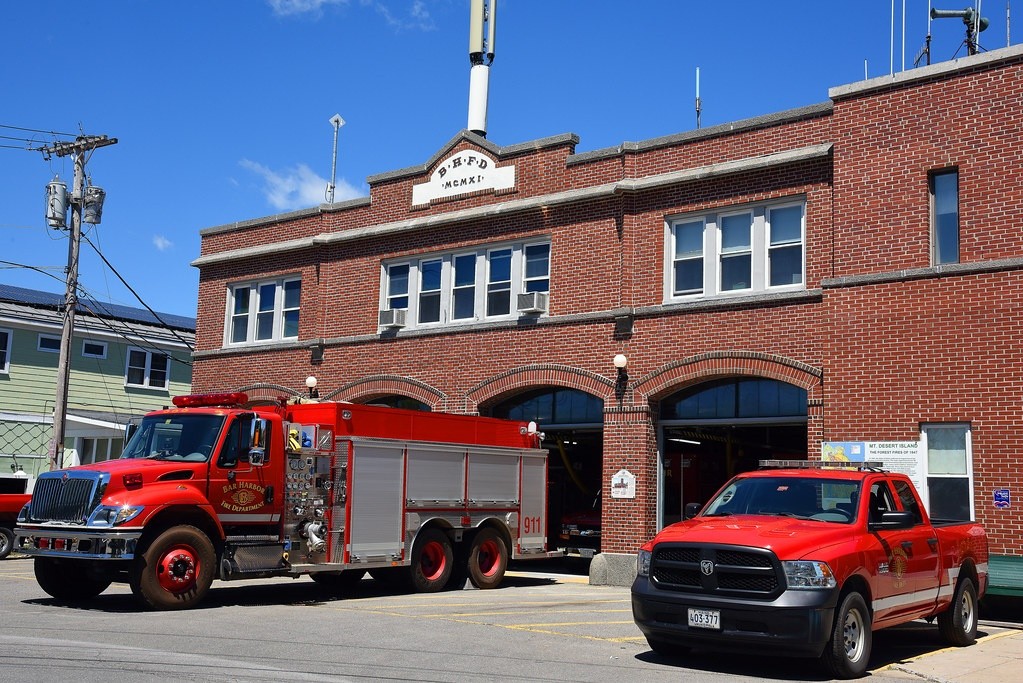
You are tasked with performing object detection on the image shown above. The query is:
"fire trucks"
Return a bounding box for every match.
[11,394,548,612]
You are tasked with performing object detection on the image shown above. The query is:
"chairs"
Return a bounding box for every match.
[851,491,882,522]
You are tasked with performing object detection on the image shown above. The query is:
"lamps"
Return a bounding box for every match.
[306,376,318,399]
[613,354,628,386]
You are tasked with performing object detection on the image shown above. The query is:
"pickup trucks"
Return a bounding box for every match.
[0,494,34,557]
[631,460,989,679]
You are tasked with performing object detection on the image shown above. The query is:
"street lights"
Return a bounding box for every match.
[306,377,318,399]
